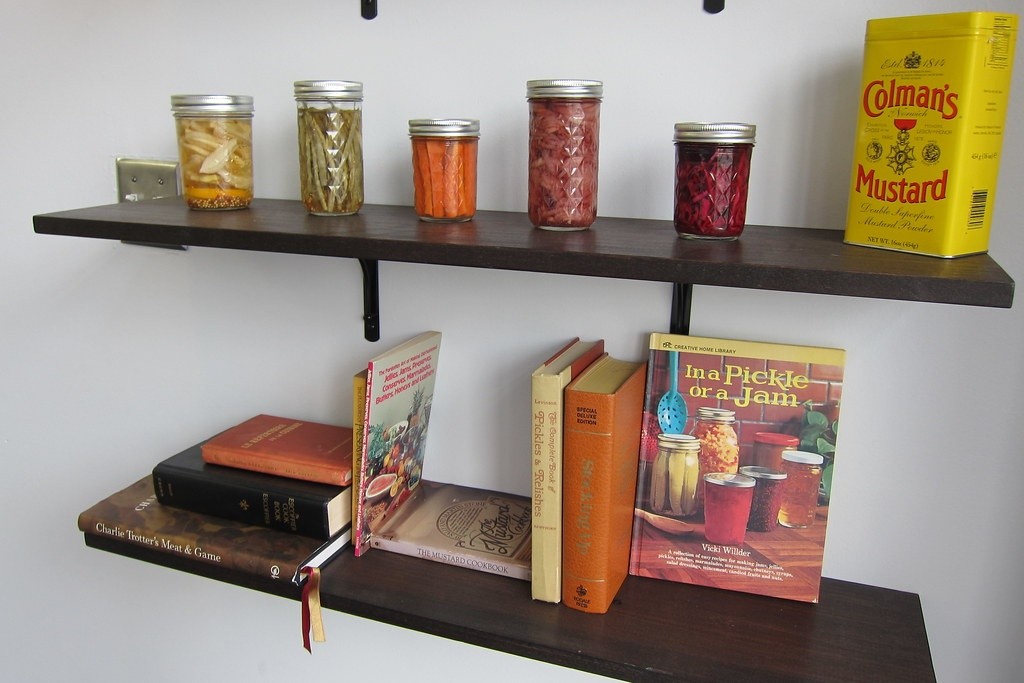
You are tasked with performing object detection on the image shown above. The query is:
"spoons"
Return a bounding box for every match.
[658,352,689,436]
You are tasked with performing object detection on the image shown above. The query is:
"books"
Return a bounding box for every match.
[531,337,648,614]
[352,331,442,557]
[78,413,353,587]
[629,332,847,604]
[371,479,532,582]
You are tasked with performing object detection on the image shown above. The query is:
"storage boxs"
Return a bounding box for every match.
[845,13,1020,259]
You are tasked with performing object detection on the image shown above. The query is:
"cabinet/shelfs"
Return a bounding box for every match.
[32,193,1015,683]
